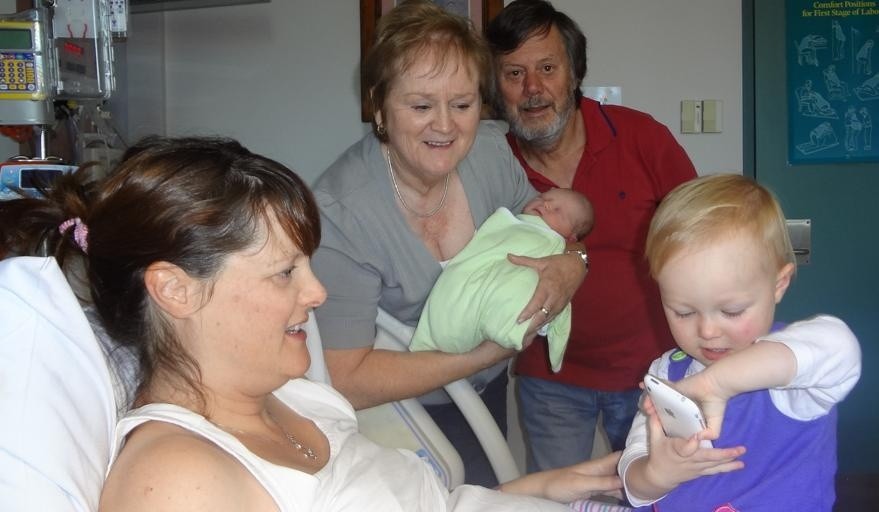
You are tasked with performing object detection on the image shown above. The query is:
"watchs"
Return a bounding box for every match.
[565,246,590,271]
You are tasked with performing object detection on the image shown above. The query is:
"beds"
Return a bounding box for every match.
[0,252,651,512]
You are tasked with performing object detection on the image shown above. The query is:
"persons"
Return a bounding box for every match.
[618,175,863,512]
[485,2,703,474]
[312,0,589,412]
[404,186,596,355]
[0,134,623,512]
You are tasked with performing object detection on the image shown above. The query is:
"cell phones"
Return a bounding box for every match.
[643,373,711,448]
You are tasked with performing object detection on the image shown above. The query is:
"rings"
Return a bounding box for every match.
[539,305,548,318]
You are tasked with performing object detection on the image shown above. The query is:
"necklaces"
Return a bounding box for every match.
[213,412,319,465]
[387,144,450,217]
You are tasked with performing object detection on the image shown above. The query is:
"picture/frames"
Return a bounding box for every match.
[358,0,507,123]
